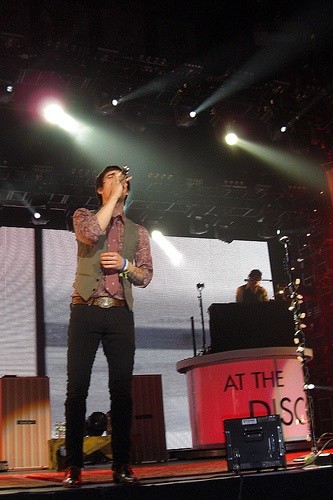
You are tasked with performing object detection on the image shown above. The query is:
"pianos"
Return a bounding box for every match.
[207,300,301,352]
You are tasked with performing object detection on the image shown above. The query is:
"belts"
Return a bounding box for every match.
[72,297,126,308]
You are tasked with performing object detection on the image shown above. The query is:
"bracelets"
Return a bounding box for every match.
[119,258,133,279]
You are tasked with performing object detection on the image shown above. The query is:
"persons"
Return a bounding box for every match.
[62,165,153,489]
[236,269,269,303]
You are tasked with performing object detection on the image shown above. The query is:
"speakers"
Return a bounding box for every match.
[224,415,286,472]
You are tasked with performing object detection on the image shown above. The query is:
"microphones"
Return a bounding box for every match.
[279,236,289,245]
[197,283,204,289]
[122,166,130,176]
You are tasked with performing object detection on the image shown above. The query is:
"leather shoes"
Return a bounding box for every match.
[63,467,82,487]
[113,470,140,483]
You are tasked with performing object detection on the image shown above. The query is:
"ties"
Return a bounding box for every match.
[105,215,120,296]
